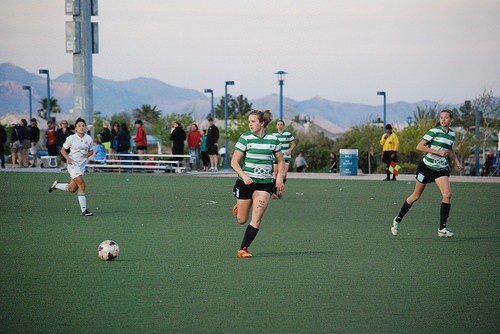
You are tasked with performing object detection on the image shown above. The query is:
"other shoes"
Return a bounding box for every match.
[61,168,67,172]
[201,168,209,171]
[391,177,396,181]
[1,165,5,168]
[278,192,283,198]
[197,168,200,170]
[385,178,390,181]
[31,163,36,168]
[271,194,278,199]
[28,162,31,165]
[209,168,218,172]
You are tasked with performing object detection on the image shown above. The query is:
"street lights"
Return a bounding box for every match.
[38,69,51,120]
[376,91,386,132]
[407,116,413,164]
[22,85,32,120]
[204,88,214,119]
[274,70,289,120]
[224,81,235,169]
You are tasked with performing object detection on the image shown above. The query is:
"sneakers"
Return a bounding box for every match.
[238,247,253,258]
[390,216,400,235]
[232,204,238,215]
[48,180,58,193]
[80,209,93,216]
[437,228,455,238]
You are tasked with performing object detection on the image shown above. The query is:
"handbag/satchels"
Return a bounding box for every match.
[12,140,22,148]
[25,143,32,148]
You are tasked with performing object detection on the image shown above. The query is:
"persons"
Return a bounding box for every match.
[45,120,90,171]
[380,124,399,181]
[230,110,286,256]
[294,151,309,173]
[451,147,500,176]
[132,120,156,172]
[271,120,296,199]
[390,109,461,237]
[329,152,337,173]
[92,120,132,173]
[0,124,7,168]
[10,118,43,168]
[169,117,226,171]
[48,117,94,216]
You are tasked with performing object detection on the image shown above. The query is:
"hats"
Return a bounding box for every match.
[386,124,392,129]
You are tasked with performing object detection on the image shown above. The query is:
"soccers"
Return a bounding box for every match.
[97,239,120,261]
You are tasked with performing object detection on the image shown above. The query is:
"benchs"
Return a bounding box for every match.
[85,154,195,173]
